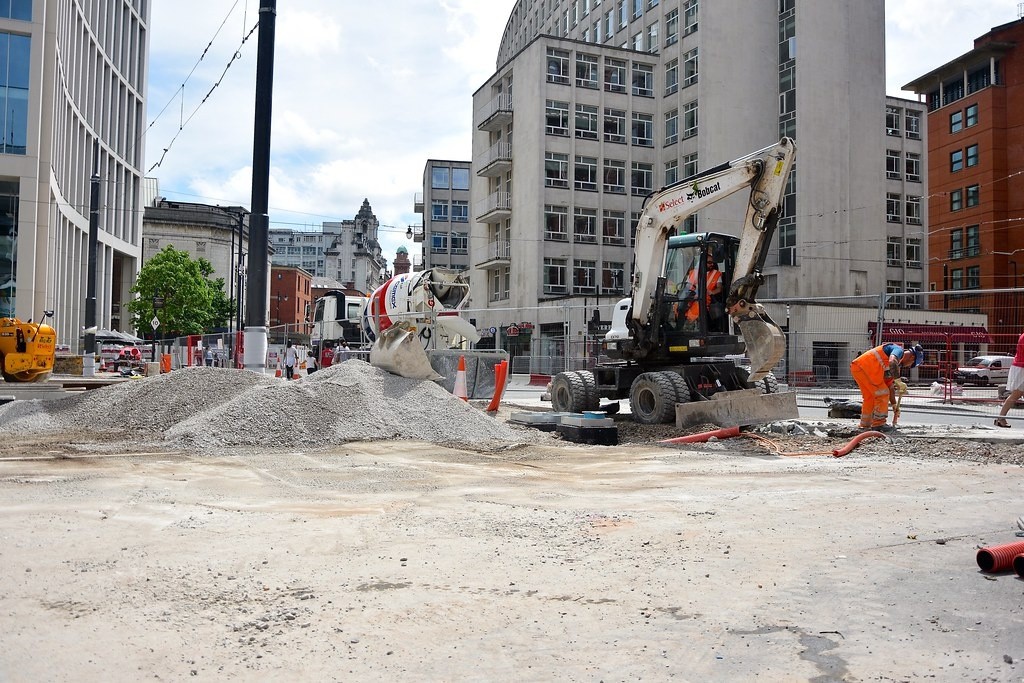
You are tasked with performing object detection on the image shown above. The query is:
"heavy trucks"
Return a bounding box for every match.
[304,266,481,370]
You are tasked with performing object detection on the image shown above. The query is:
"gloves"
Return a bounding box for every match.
[892,404,900,417]
[895,378,907,394]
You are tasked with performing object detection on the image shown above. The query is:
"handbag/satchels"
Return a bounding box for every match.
[336,351,341,362]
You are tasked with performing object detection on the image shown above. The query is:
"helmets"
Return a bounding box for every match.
[910,346,924,368]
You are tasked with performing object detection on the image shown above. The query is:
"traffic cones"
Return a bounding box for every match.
[291,365,299,379]
[275,358,282,377]
[450,355,467,403]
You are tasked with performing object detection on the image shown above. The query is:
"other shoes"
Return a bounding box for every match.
[871,424,893,431]
[997,419,1011,427]
[858,427,871,431]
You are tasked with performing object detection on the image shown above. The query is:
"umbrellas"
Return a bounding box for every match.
[81,328,144,343]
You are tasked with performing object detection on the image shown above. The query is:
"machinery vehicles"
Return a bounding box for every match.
[0,310,57,382]
[551,137,799,426]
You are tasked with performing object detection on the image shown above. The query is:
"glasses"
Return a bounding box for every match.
[708,260,715,265]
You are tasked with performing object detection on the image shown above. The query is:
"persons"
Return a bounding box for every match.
[673,255,722,328]
[994,333,1024,428]
[284,342,297,380]
[850,342,923,431]
[300,352,319,375]
[336,339,351,363]
[322,343,334,369]
[203,345,213,367]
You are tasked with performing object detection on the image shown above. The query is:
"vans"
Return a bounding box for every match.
[954,355,1014,387]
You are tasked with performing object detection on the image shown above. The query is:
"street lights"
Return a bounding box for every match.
[277,287,289,344]
[217,223,245,360]
[222,208,269,369]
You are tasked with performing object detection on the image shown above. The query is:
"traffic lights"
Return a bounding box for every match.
[592,310,600,328]
